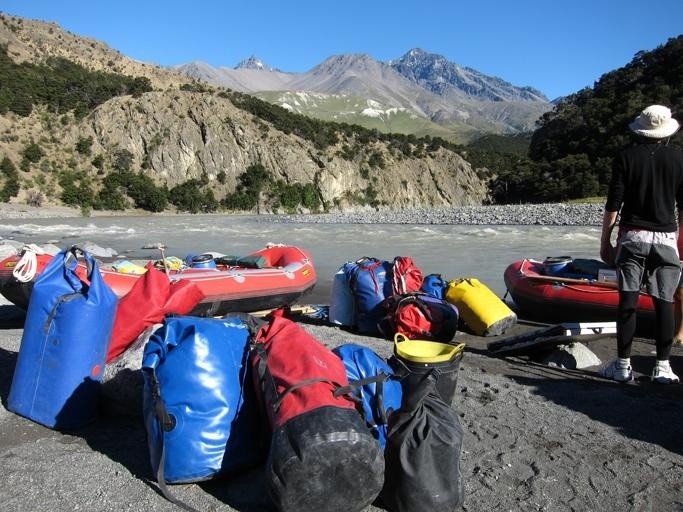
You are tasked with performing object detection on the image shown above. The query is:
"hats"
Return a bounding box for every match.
[628,105,681,139]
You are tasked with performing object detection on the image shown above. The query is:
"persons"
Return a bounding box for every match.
[597,102,683,387]
[669,224,683,345]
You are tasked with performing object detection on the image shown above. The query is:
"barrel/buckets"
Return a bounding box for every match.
[392,332,464,405]
[543,256,574,275]
[191,253,217,269]
[598,268,616,282]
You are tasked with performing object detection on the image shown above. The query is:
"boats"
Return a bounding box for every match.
[0,243,316,318]
[503,256,681,341]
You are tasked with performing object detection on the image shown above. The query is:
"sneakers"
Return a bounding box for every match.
[599,361,633,382]
[651,366,680,385]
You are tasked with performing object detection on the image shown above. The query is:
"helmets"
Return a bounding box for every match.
[542,255,571,276]
